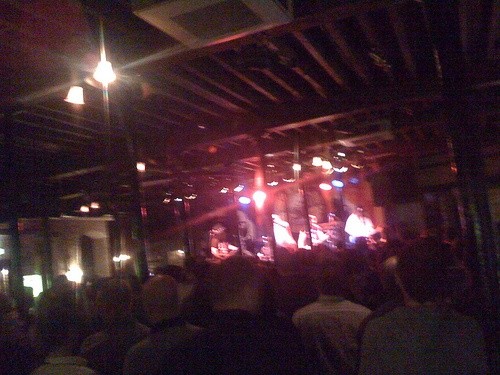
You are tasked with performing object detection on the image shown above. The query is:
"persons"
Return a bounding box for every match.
[0,205,500,375]
